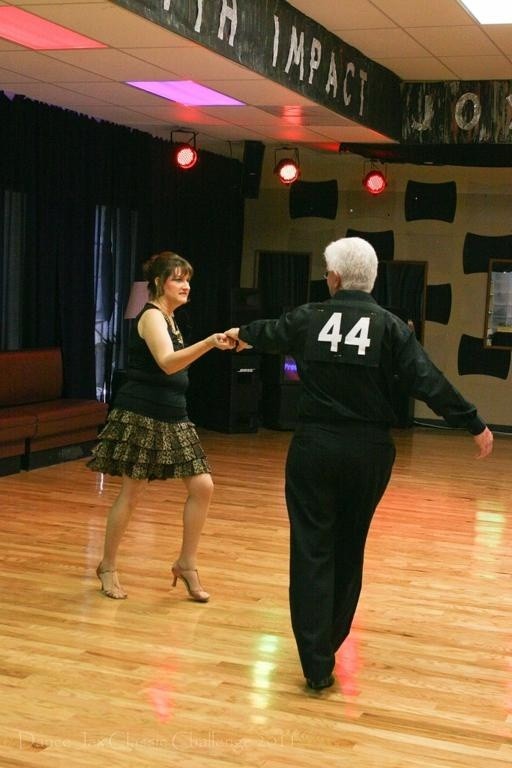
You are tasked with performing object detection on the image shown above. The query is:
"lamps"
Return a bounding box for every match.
[171,127,202,172]
[361,154,390,195]
[273,145,303,187]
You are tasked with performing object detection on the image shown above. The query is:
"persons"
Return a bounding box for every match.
[224,237,496,689]
[87,248,237,602]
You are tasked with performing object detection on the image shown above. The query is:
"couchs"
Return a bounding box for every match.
[0,345,106,477]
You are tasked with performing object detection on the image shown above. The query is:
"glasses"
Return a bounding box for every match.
[323,271,328,279]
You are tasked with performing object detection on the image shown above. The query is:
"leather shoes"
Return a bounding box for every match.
[308,676,335,690]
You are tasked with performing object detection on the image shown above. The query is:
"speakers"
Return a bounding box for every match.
[257,351,302,431]
[215,162,260,200]
[196,352,262,434]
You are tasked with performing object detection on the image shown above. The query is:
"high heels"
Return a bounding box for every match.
[171,562,211,602]
[97,561,127,598]
